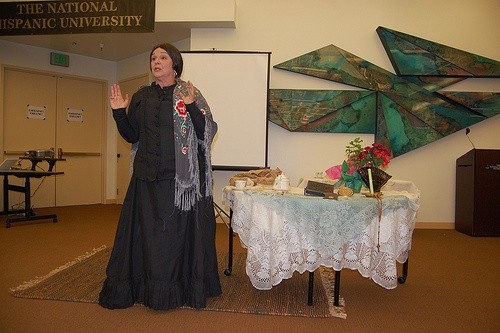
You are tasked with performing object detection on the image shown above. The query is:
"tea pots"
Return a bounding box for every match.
[274,172,290,190]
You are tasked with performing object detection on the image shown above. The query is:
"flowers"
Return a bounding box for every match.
[346,138,391,174]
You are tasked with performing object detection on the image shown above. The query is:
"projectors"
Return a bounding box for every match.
[27,149,52,157]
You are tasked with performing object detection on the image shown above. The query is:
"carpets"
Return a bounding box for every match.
[8,244,348,319]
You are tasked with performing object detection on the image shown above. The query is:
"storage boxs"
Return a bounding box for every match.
[36,149,52,158]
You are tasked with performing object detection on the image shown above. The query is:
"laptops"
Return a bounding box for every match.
[0,158,23,167]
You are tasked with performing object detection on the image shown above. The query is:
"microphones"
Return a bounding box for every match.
[466,128,476,148]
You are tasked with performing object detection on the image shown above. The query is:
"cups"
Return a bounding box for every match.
[236,180,246,188]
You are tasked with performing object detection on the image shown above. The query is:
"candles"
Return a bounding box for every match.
[368,168,373,195]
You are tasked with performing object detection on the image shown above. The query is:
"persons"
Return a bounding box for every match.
[99,43,223,311]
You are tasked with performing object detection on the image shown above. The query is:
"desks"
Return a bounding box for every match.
[222,180,420,307]
[0,157,66,229]
